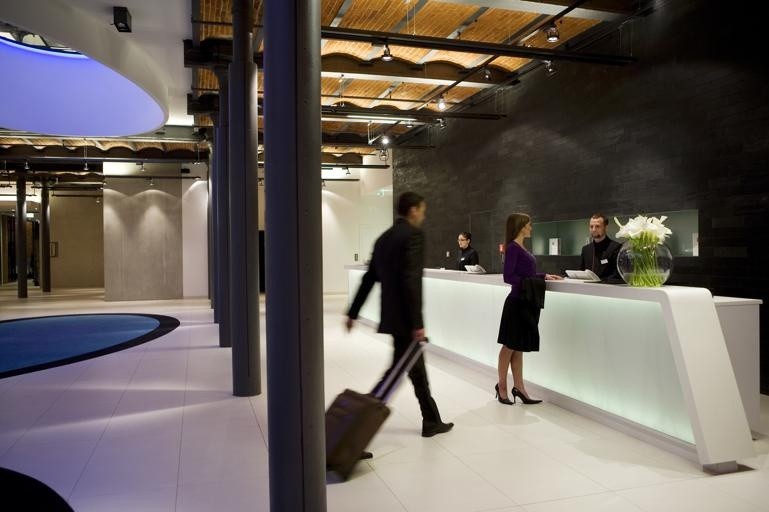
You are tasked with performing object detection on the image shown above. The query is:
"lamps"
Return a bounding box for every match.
[546,21,561,44]
[381,43,394,63]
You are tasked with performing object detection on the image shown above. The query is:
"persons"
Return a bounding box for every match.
[453,232,479,270]
[580,213,629,279]
[495,213,563,404]
[345,193,454,459]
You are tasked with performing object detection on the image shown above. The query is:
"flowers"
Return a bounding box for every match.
[612,214,673,289]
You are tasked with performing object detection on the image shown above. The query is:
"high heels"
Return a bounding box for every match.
[511,387,543,405]
[493,383,514,405]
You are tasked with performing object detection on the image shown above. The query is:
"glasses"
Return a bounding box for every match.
[457,239,467,241]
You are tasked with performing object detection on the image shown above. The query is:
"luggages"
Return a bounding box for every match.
[326,389,391,481]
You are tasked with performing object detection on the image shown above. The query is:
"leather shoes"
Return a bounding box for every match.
[421,422,454,438]
[359,451,373,460]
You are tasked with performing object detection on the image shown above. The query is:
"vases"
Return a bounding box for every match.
[616,239,673,290]
